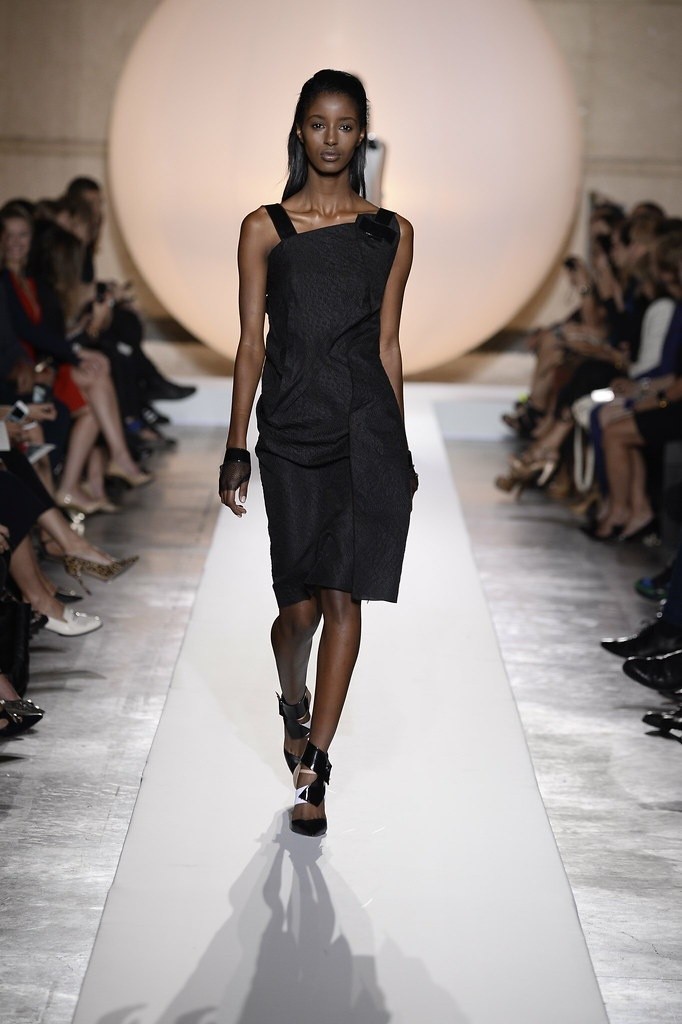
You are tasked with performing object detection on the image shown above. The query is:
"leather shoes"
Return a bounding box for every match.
[0,698,46,737]
[600,599,682,657]
[642,699,682,730]
[624,649,682,694]
[635,577,682,598]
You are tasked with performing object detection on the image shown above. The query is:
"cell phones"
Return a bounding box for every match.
[96,283,106,303]
[4,400,30,425]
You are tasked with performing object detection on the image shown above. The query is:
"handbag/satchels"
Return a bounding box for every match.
[573,392,629,428]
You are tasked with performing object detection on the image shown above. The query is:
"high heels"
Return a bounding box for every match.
[106,459,152,487]
[81,483,118,514]
[63,554,140,596]
[53,489,107,523]
[276,689,312,772]
[293,740,332,836]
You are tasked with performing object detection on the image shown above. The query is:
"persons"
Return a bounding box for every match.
[496,192,682,733]
[219,68,419,837]
[0,176,197,738]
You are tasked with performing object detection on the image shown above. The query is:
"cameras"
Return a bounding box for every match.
[565,258,578,271]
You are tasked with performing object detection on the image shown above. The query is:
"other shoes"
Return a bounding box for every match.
[494,396,660,549]
[43,605,103,637]
[128,423,165,449]
[150,381,196,399]
[38,530,65,564]
[141,407,169,426]
[55,584,83,604]
